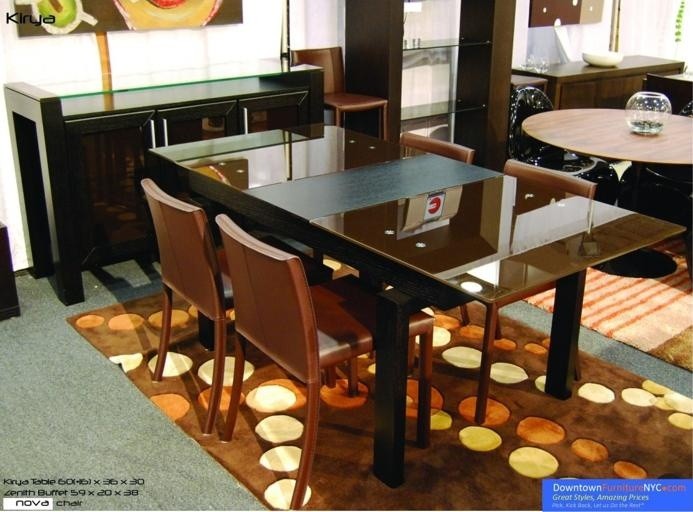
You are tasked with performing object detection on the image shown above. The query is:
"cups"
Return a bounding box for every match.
[535,56,552,73]
[521,54,536,71]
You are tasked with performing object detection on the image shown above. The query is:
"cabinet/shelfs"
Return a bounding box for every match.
[0,59,322,307]
[403,38,492,126]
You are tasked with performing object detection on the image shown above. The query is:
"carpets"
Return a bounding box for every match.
[63,226,692,511]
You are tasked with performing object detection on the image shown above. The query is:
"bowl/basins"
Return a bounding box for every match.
[582,51,624,68]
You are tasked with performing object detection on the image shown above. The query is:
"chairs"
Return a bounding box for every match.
[137,85,619,509]
[288,44,390,142]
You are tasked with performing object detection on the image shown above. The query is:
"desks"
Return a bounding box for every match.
[512,54,685,109]
[146,103,692,487]
